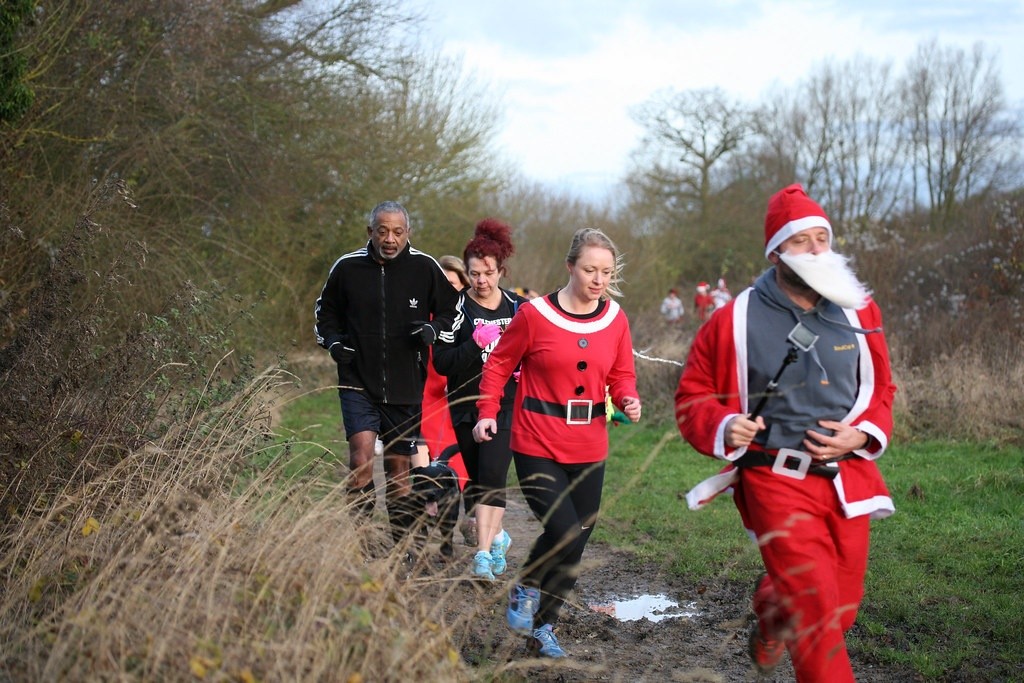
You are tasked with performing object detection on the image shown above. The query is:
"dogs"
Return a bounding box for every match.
[384,464,461,561]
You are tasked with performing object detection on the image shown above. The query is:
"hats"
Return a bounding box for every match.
[695,282,713,292]
[761,184,838,259]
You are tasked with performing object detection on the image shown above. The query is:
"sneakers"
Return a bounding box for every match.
[506,583,543,637]
[526,623,569,658]
[491,530,513,576]
[472,552,498,583]
[749,570,789,670]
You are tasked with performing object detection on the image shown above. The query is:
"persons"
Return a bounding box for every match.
[314,200,459,552]
[674,184,895,683]
[693,278,731,320]
[431,218,530,584]
[473,229,641,656]
[661,290,683,332]
[410,252,476,546]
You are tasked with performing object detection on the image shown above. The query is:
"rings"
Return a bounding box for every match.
[823,454,826,460]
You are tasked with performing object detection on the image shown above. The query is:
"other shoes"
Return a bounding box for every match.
[442,534,456,558]
[396,548,426,574]
[359,483,380,511]
[460,518,482,546]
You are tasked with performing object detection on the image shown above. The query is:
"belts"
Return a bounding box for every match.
[731,449,858,480]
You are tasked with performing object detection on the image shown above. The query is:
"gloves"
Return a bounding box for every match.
[328,342,361,365]
[410,320,448,351]
[472,323,501,349]
[514,363,523,384]
